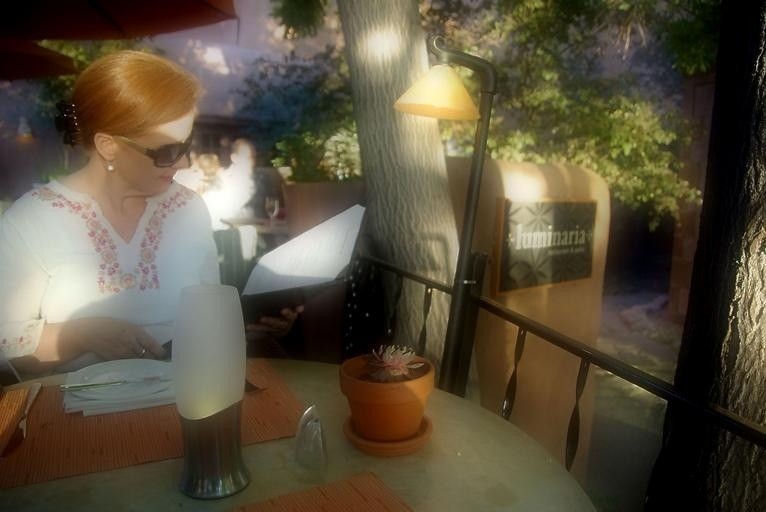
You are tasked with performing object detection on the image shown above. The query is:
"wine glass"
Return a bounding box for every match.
[265,196,279,229]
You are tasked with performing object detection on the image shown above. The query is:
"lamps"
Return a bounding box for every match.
[392,33,497,391]
[173,284,250,499]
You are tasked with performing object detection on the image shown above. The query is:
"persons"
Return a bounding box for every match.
[0,47,307,386]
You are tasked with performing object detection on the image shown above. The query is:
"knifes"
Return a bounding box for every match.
[1,383,43,459]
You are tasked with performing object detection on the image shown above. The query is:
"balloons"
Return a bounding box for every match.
[153,196,372,353]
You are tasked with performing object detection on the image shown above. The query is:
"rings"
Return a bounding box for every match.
[136,344,147,356]
[278,323,287,330]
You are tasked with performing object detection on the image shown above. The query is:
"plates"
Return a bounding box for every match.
[67,358,175,400]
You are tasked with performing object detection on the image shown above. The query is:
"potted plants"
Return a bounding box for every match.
[341,343,435,442]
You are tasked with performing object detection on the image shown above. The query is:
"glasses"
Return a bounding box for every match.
[114,131,193,168]
